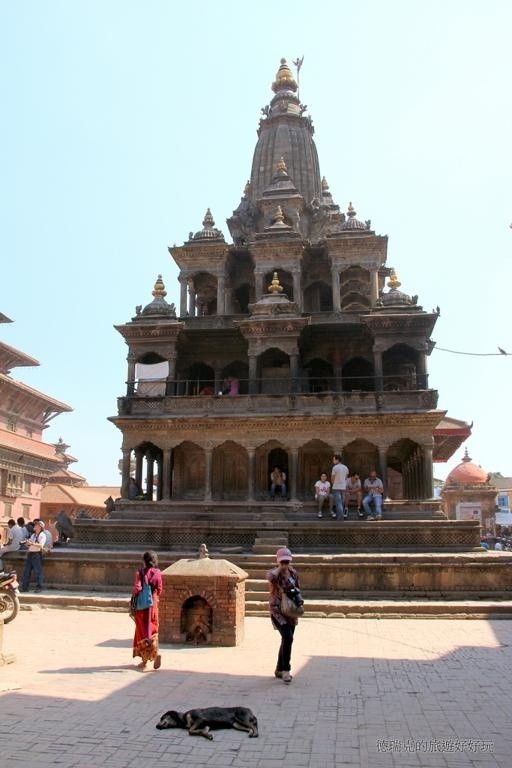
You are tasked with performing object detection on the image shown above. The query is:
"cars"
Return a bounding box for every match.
[479,534,512,545]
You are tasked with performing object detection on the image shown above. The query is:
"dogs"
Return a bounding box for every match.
[156,706,258,740]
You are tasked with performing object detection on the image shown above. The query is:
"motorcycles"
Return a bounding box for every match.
[0,566,22,625]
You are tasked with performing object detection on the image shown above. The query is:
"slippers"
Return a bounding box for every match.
[154,655,161,669]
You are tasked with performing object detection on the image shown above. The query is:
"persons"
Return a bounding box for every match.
[131,478,142,498]
[479,536,512,552]
[200,383,215,396]
[264,548,305,683]
[314,454,384,521]
[269,466,288,500]
[128,550,163,671]
[18,520,47,593]
[226,374,239,395]
[0,516,53,572]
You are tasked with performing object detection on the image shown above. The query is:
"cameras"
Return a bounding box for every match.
[289,587,303,607]
[19,539,31,546]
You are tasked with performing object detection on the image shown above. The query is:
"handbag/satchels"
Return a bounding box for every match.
[131,567,154,611]
[277,585,305,619]
[40,546,51,558]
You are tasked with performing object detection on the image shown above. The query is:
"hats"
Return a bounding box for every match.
[276,547,293,563]
[34,521,45,531]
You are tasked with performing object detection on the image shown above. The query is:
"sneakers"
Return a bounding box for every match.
[330,510,334,513]
[343,509,348,517]
[366,516,375,521]
[34,586,42,592]
[275,670,292,682]
[18,587,28,592]
[317,511,323,517]
[375,514,385,521]
[357,511,365,517]
